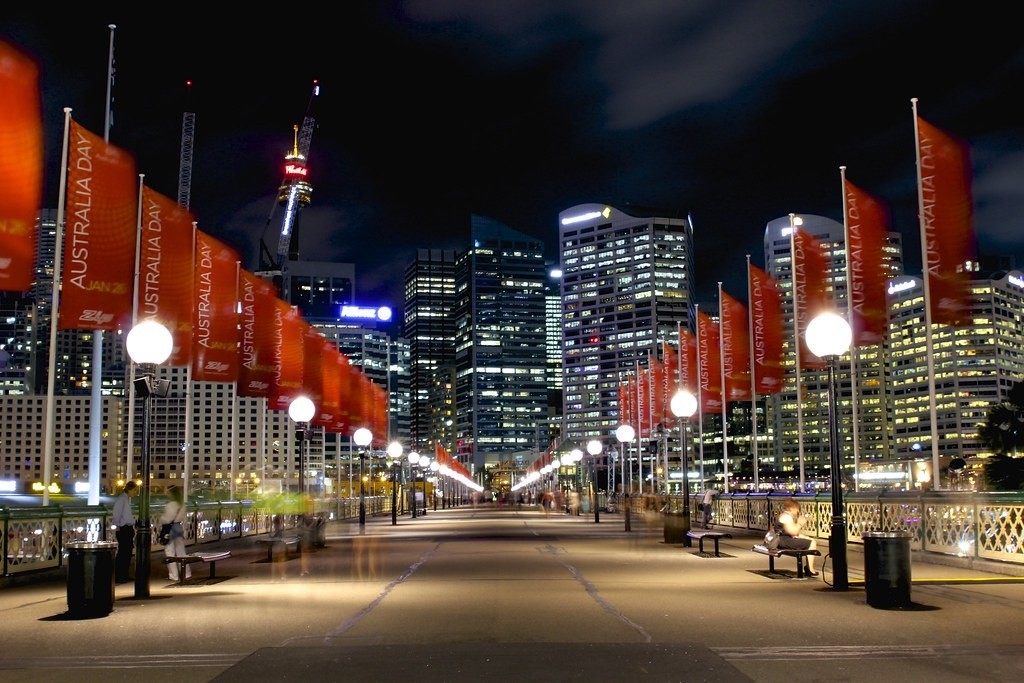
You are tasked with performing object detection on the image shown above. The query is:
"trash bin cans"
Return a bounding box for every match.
[63,540,118,615]
[664,510,684,542]
[860,530,914,607]
[306,516,326,546]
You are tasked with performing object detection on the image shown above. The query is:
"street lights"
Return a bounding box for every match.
[289,396,315,495]
[407,452,419,518]
[385,441,403,526]
[354,428,372,535]
[420,456,430,515]
[616,424,636,532]
[126,320,173,600]
[561,454,573,513]
[570,449,584,494]
[440,465,485,509]
[805,311,854,591]
[430,461,439,511]
[587,439,602,523]
[669,390,698,546]
[509,459,561,513]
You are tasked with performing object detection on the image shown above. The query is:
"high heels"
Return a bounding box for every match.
[804,565,819,577]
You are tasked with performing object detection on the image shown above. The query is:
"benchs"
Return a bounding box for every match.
[162,551,232,585]
[752,543,821,581]
[687,530,732,558]
[257,534,304,562]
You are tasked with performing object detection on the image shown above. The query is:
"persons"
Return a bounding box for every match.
[700,489,719,530]
[497,492,588,515]
[608,496,617,509]
[112,481,137,581]
[160,485,191,580]
[773,500,820,578]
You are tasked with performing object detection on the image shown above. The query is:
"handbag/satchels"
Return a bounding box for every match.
[160,521,173,545]
[764,531,780,550]
[698,503,704,511]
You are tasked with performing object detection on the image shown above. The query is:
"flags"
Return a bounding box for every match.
[620,116,972,436]
[437,443,470,478]
[0,42,385,445]
